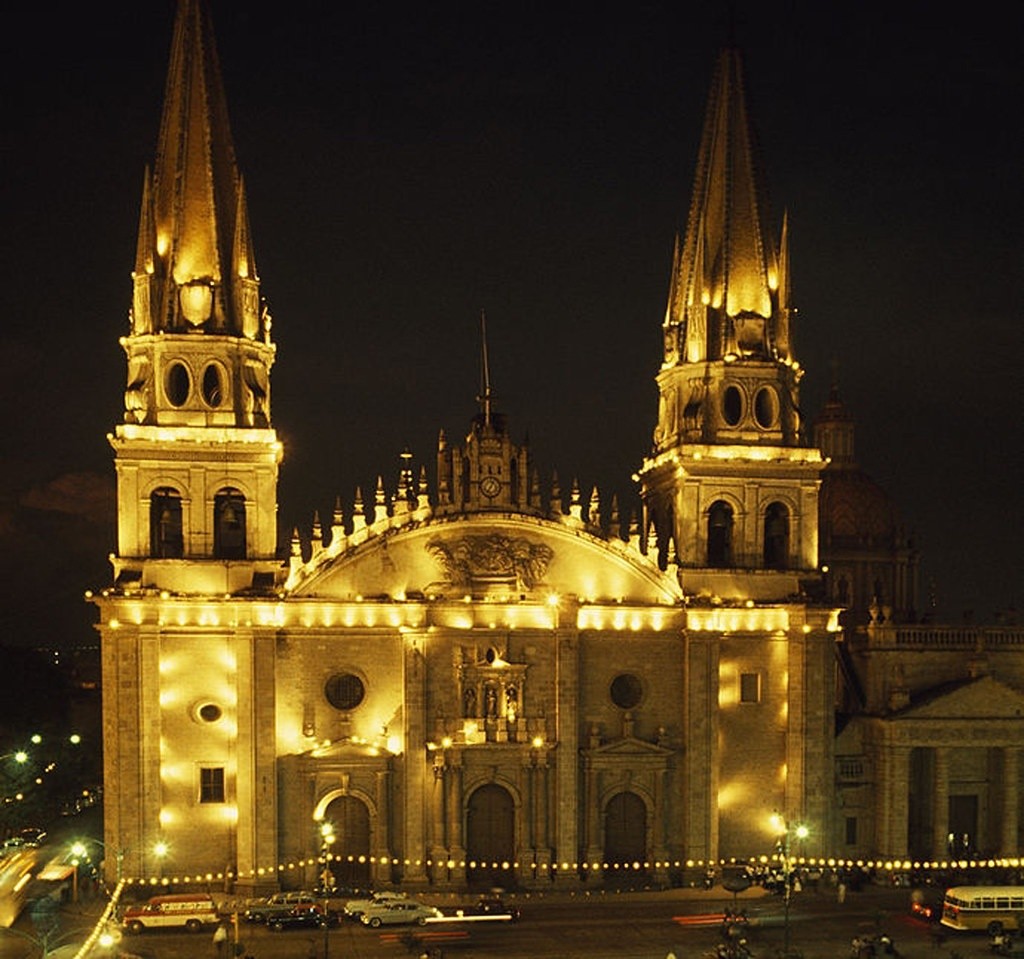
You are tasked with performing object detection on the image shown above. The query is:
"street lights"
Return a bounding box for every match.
[771,814,809,951]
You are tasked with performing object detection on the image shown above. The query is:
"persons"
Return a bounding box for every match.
[487,690,496,717]
[706,868,715,891]
[507,689,516,718]
[464,688,476,718]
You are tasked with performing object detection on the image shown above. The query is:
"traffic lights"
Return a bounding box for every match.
[230,910,239,924]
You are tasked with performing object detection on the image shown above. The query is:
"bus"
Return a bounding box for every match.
[939,885,1024,937]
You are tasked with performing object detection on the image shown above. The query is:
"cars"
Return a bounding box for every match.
[343,891,407,922]
[456,898,520,925]
[361,899,446,928]
[266,903,342,931]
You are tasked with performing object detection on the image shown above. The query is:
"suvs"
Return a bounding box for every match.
[244,890,318,924]
[122,892,219,936]
